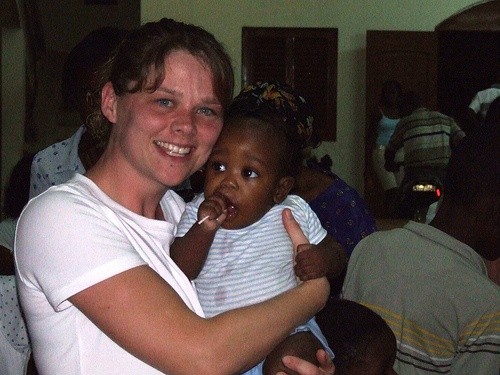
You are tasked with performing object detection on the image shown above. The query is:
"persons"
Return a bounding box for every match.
[312,297,399,375]
[0,151,35,276]
[28,26,128,202]
[286,155,376,265]
[383,85,466,223]
[338,125,500,375]
[363,79,413,219]
[169,80,347,375]
[12,16,336,375]
